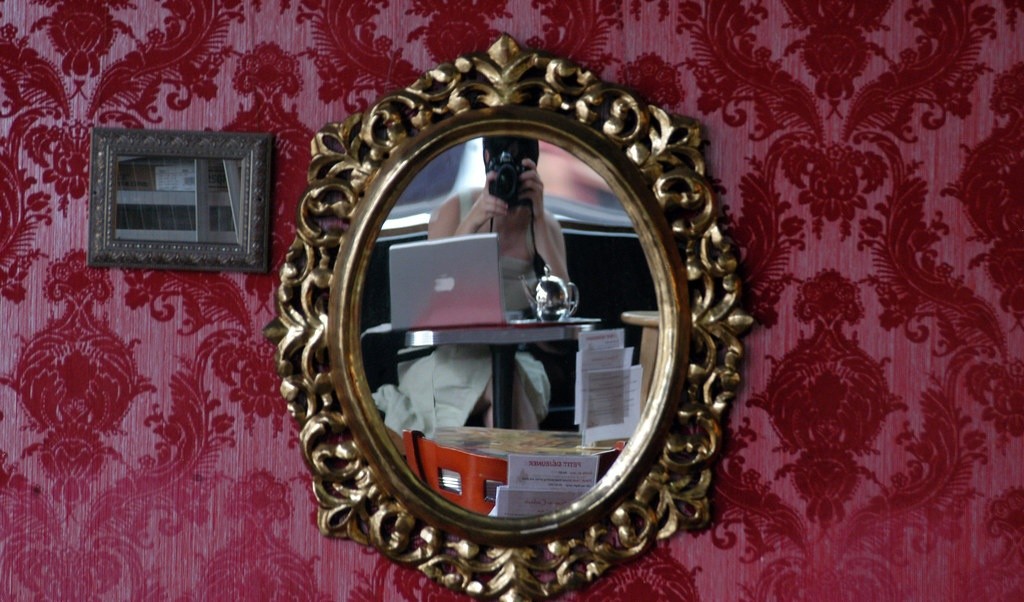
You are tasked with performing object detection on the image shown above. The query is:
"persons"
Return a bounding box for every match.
[398,130,569,431]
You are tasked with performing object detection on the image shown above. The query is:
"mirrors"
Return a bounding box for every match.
[259,35,753,602]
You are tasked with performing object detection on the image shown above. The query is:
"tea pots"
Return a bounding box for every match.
[519,266,579,321]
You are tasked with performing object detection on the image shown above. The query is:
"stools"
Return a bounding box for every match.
[621,311,660,416]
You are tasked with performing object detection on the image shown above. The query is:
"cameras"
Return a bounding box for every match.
[487,150,533,208]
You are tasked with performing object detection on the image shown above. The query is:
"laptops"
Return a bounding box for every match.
[389,233,602,330]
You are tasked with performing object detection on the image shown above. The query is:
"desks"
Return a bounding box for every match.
[401,319,596,429]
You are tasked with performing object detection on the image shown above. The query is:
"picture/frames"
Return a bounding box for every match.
[87,128,275,274]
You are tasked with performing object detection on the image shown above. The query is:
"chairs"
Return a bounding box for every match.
[403,429,628,518]
